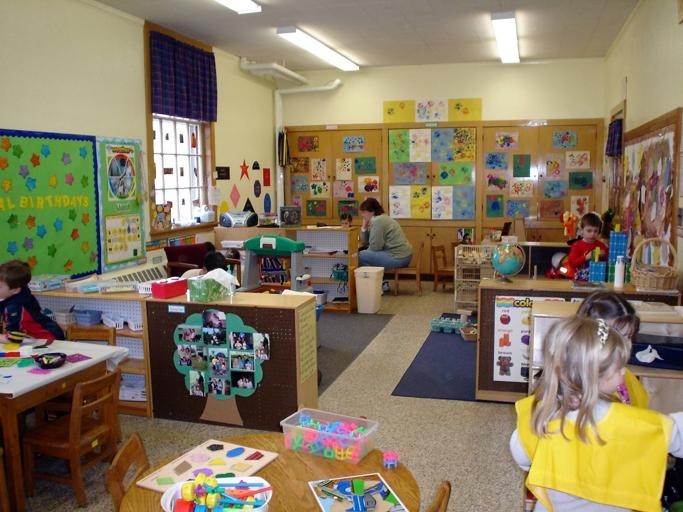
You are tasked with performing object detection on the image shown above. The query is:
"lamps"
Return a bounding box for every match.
[213,0,263,15]
[276,26,359,72]
[490,11,520,64]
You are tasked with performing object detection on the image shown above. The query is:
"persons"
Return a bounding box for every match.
[178,311,270,398]
[357,197,412,295]
[0,259,64,342]
[569,213,608,270]
[576,290,659,410]
[180,251,238,289]
[508,315,682,512]
[338,213,351,226]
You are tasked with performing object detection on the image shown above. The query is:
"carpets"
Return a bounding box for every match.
[393,312,515,403]
[315,314,395,396]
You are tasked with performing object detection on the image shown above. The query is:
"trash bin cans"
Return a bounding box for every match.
[354,266,385,314]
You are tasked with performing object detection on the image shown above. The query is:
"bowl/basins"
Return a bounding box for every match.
[34,352,66,369]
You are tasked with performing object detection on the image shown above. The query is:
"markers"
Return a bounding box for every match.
[1,352,20,357]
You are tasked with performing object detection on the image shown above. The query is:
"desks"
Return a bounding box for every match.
[285,226,358,314]
[358,239,366,250]
[1,335,130,511]
[120,431,421,512]
[454,244,498,308]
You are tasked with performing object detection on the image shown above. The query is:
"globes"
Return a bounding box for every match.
[492,243,524,276]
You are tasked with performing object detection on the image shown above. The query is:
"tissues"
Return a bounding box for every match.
[187,267,241,303]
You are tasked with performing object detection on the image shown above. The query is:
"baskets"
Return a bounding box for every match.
[102,313,124,330]
[137,280,153,294]
[55,312,76,325]
[460,326,477,341]
[127,319,143,331]
[74,309,102,325]
[629,237,678,291]
[46,313,56,320]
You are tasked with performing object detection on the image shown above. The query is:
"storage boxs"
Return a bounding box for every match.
[279,406,380,465]
[628,343,683,370]
[333,269,348,281]
[258,214,278,226]
[311,288,327,306]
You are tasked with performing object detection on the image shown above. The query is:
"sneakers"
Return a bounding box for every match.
[382,280,390,291]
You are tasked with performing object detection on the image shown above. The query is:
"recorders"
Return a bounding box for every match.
[220,210,258,227]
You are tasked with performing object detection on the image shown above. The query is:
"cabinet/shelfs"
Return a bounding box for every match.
[30,286,150,418]
[480,241,572,278]
[146,291,316,433]
[476,279,679,401]
[527,300,683,401]
[214,225,288,249]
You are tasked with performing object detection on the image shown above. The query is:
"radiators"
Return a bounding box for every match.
[99,261,167,286]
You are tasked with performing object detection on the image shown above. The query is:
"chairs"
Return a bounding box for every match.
[68,325,116,345]
[31,369,122,506]
[163,241,242,289]
[426,480,451,512]
[106,432,150,511]
[431,244,455,292]
[394,242,424,295]
